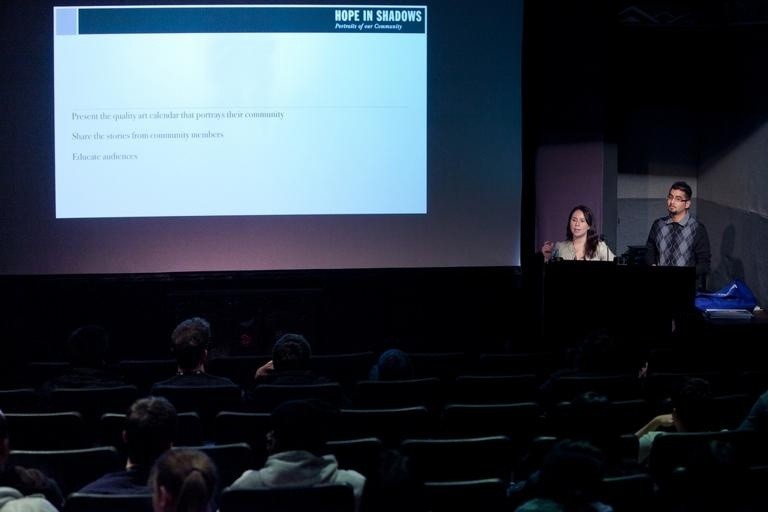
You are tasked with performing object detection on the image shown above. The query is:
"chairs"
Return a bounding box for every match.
[1,341,768,511]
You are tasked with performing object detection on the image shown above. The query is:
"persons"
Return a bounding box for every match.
[503,439,612,510]
[642,181,712,280]
[1,316,413,509]
[633,381,768,466]
[540,206,616,263]
[542,336,672,399]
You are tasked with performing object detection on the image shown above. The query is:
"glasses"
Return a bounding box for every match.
[666,193,689,203]
